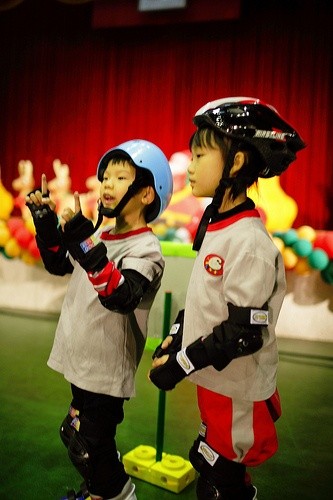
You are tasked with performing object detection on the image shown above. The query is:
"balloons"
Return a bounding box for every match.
[145,218,194,247]
[268,225,333,279]
[0,179,45,266]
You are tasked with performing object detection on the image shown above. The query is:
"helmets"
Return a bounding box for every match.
[97,138,174,223]
[194,96,305,178]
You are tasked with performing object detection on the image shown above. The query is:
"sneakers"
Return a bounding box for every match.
[64,450,137,500]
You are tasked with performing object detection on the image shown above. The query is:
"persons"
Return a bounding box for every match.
[153,96,307,500]
[20,137,173,500]
